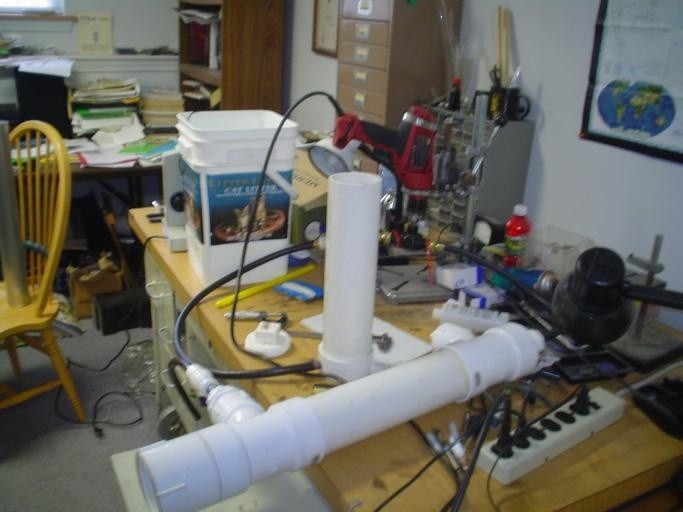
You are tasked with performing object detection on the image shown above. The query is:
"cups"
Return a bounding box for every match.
[489,87,530,123]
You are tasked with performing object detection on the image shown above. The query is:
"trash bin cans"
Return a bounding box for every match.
[65,262,124,319]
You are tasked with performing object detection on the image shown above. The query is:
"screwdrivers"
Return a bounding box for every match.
[225,312,284,320]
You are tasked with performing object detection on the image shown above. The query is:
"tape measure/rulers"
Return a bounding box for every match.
[496,6,510,88]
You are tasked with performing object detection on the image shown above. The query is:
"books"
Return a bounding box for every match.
[172,7,220,69]
[10,77,186,172]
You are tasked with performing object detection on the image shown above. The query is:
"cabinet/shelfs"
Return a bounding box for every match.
[177,1,283,114]
[336,1,464,177]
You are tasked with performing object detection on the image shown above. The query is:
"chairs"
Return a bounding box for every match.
[0,118,88,426]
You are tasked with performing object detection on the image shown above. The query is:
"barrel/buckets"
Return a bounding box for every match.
[176,110,298,287]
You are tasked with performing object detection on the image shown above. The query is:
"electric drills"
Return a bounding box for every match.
[332,104,438,248]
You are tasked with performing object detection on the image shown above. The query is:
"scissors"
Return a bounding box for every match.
[486,68,531,127]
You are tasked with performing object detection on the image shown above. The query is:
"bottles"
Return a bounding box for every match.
[505,203,531,265]
[448,77,461,111]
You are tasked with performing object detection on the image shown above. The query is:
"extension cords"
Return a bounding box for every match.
[476,386,626,485]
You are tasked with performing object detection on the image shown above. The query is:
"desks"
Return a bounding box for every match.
[127,206,682,512]
[12,157,163,279]
